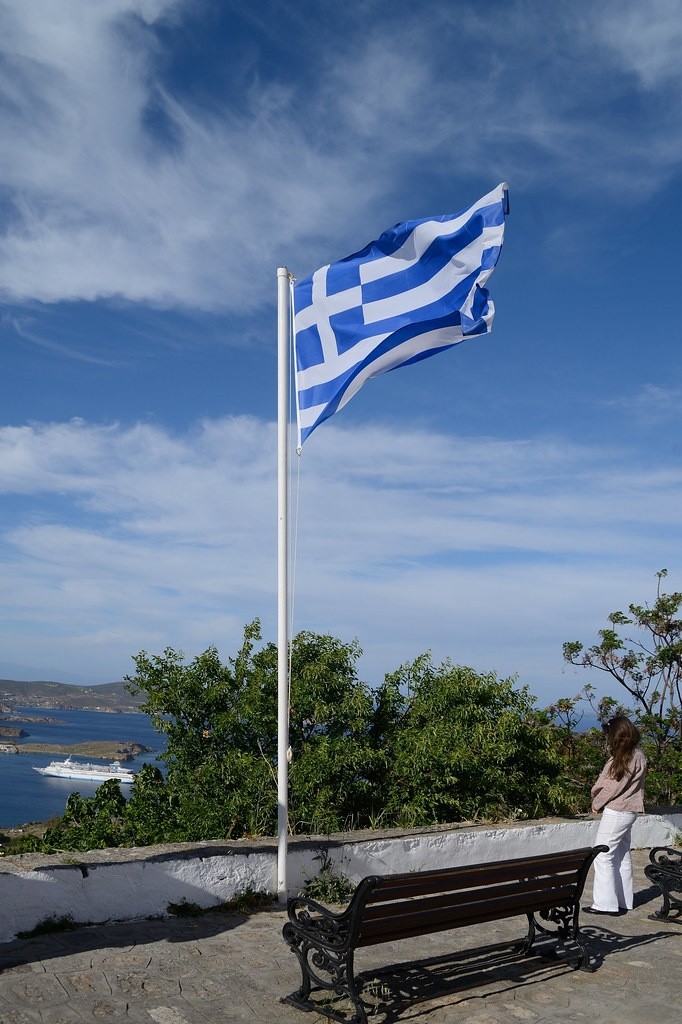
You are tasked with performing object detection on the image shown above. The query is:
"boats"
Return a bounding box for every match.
[32,754,136,783]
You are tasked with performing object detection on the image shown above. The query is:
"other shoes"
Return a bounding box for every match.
[581,906,617,916]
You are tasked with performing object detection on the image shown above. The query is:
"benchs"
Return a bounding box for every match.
[644,847,682,925]
[278,845,610,1024]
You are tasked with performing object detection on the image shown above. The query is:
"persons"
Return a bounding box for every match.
[581,717,647,916]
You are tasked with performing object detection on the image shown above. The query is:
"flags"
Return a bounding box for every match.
[291,183,510,442]
